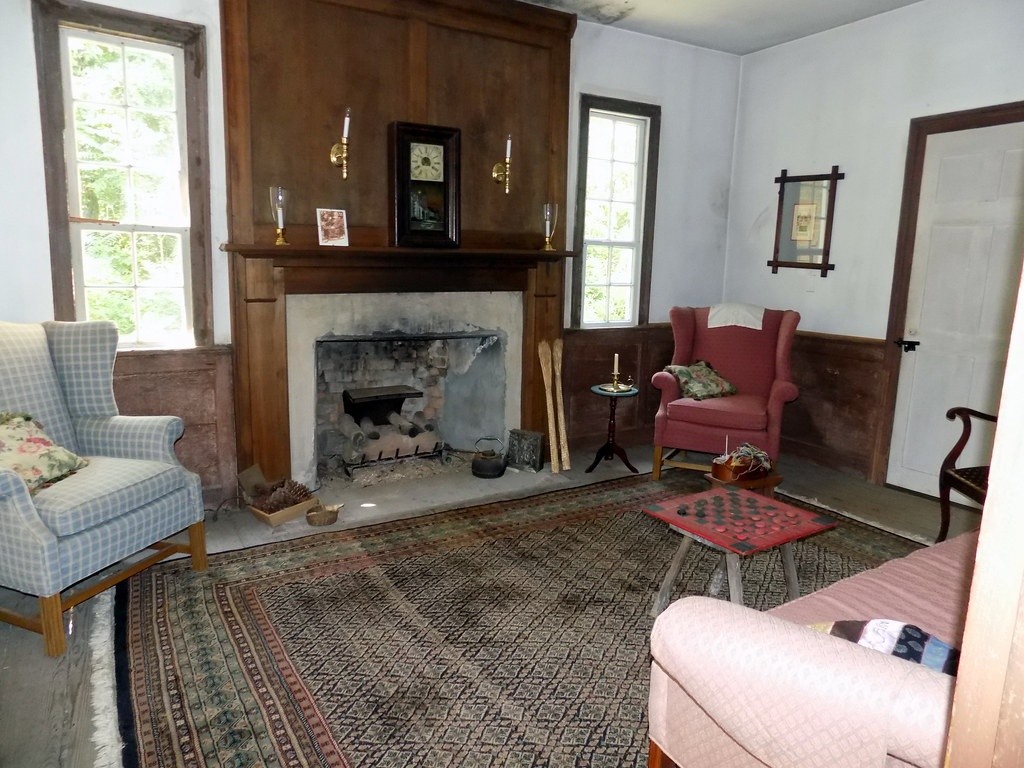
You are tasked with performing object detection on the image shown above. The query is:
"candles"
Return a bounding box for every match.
[614,354,618,373]
[506,140,511,158]
[277,208,284,227]
[545,221,551,238]
[342,116,351,138]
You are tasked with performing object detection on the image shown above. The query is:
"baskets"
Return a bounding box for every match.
[306,497,344,526]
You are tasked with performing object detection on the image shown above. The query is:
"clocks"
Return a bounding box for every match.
[388,122,460,248]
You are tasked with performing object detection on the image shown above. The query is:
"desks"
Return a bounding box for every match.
[584,384,639,474]
[650,523,800,616]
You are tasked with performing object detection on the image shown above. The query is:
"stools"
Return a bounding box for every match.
[705,473,784,500]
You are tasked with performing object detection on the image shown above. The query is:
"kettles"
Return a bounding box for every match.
[471,437,518,478]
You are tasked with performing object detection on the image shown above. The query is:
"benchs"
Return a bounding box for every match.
[648,529,986,768]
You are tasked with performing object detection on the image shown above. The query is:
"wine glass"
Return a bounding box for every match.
[538,202,558,252]
[269,187,291,245]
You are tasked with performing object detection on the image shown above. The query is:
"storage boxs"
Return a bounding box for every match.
[248,495,318,527]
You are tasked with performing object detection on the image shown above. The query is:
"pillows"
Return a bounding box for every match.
[662,361,737,400]
[0,412,88,494]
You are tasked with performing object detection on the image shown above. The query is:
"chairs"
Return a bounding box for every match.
[0,322,213,656]
[934,408,999,544]
[652,305,797,487]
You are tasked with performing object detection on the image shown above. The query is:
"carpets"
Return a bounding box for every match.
[114,463,928,768]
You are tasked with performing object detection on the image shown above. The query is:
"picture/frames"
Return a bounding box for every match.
[316,208,349,246]
